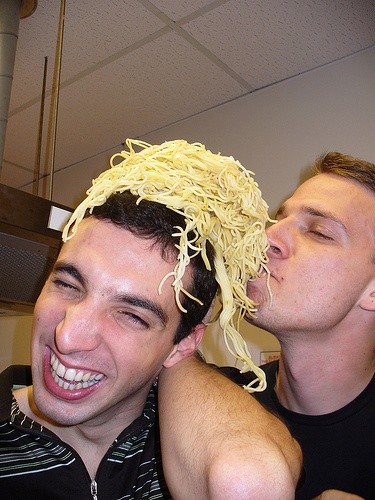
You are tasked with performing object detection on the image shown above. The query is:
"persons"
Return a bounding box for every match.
[205,151,375,500]
[0,189,303,500]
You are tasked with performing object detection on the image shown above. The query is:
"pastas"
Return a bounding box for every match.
[63,138,278,393]
